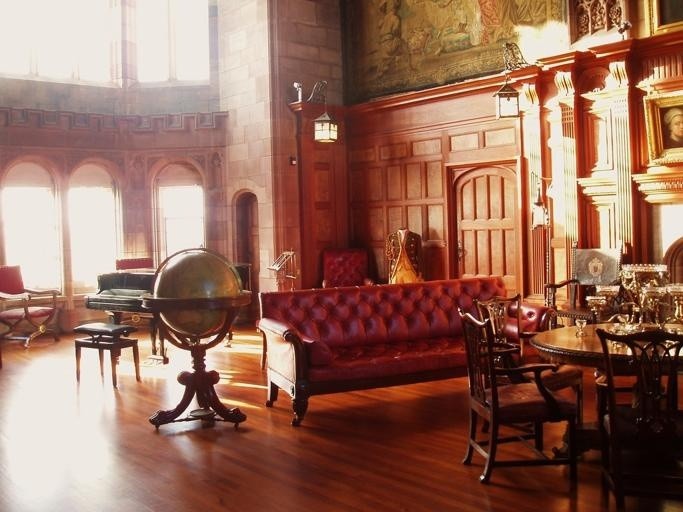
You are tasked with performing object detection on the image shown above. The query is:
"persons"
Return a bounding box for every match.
[383,227,424,285]
[660,107,682,148]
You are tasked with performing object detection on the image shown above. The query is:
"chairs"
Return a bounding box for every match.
[113,255,155,325]
[0,264,62,351]
[319,248,378,291]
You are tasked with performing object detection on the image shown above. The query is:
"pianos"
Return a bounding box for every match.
[84,269,168,363]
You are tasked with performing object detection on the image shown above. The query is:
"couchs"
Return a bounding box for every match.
[251,275,558,423]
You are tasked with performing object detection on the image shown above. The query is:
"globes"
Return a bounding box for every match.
[140,248,252,431]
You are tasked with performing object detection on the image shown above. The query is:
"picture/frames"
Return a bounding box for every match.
[639,95,682,168]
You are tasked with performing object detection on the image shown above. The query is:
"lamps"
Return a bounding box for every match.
[291,77,343,145]
[491,40,532,120]
[530,174,552,231]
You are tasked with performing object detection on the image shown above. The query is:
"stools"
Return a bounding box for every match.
[73,320,143,390]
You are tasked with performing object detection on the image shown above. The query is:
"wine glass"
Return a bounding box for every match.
[576,319,586,337]
